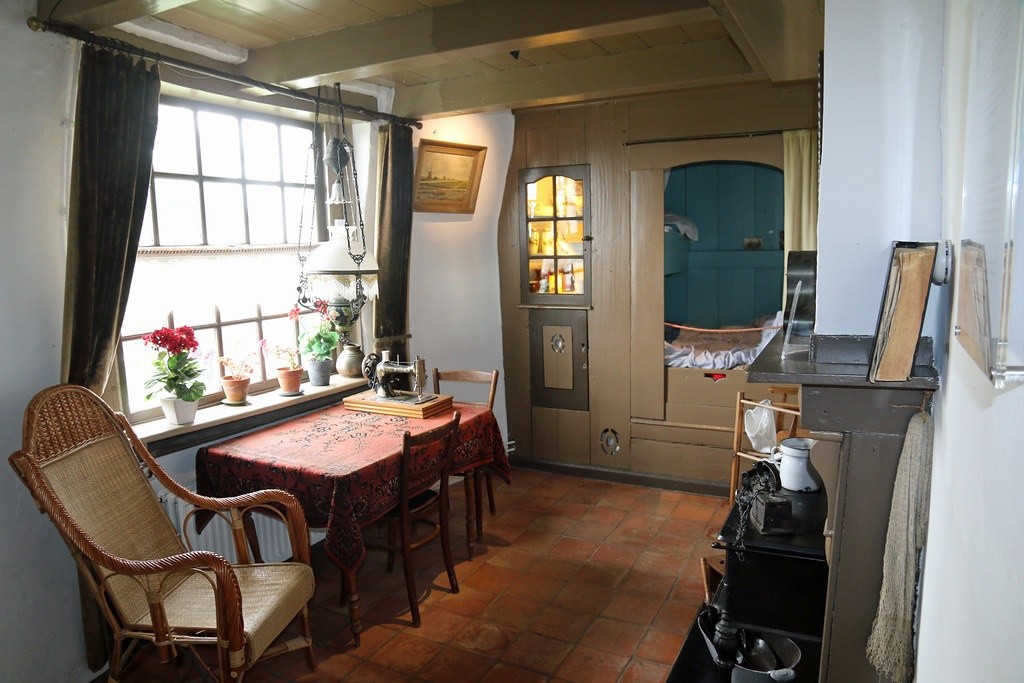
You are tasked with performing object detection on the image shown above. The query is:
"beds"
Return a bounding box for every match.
[666,312,784,382]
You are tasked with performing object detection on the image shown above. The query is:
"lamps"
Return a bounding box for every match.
[297,83,383,346]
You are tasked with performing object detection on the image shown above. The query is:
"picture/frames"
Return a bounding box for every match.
[411,138,487,214]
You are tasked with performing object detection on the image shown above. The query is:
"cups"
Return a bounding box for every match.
[769,436,825,493]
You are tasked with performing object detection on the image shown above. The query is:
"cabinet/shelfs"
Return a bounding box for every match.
[710,485,829,659]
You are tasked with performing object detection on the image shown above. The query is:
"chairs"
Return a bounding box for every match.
[432,366,500,559]
[7,382,319,683]
[339,409,462,629]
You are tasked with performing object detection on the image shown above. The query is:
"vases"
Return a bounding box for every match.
[221,375,251,405]
[162,396,200,425]
[307,358,332,386]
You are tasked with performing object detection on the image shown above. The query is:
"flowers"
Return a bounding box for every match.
[144,325,207,402]
[218,339,266,379]
[288,295,340,362]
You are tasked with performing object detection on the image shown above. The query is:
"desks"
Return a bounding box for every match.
[196,399,514,644]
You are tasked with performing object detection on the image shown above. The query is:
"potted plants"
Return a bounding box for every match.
[272,344,306,397]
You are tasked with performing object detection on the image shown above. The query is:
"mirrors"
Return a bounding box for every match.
[955,77,1024,391]
